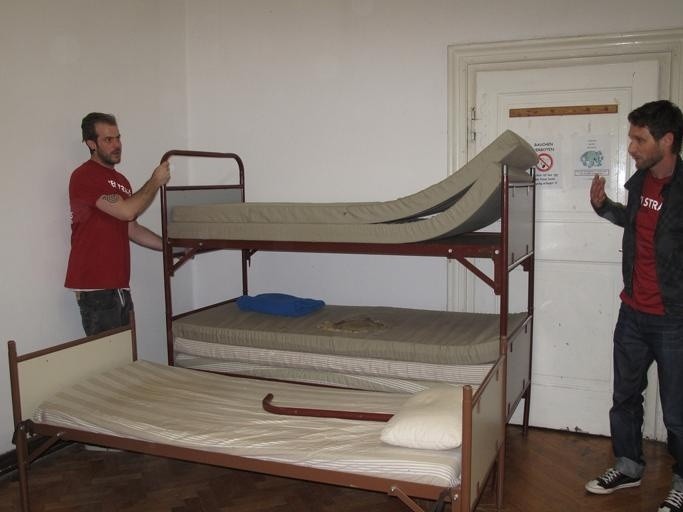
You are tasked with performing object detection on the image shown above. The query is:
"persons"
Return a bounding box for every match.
[581,100,683,511]
[63,112,194,454]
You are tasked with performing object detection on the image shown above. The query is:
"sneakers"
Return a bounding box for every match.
[82,442,125,453]
[585,467,642,495]
[657,487,683,512]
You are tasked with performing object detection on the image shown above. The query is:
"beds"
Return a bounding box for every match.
[160,150,536,438]
[9,311,507,511]
